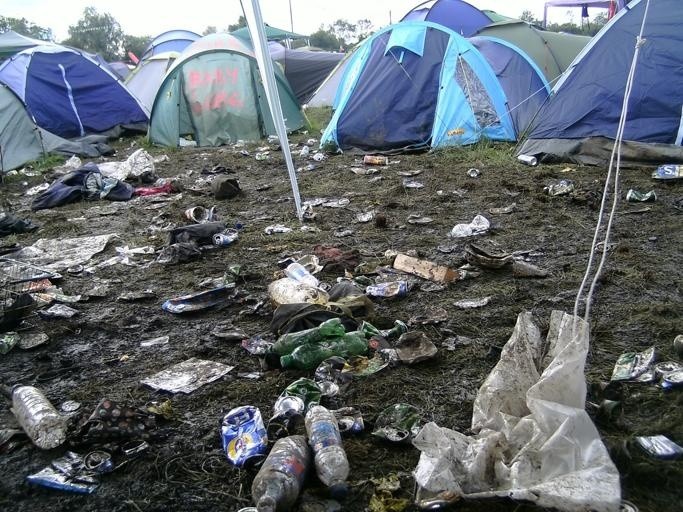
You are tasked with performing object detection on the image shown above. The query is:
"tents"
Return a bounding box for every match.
[308,1,683,162]
[1,23,350,173]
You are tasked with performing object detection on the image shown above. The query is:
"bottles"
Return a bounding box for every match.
[286,263,319,287]
[251,317,369,512]
[11,382,67,449]
[446,214,490,238]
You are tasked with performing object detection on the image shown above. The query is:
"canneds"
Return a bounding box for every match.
[222,405,268,469]
[85,438,151,472]
[1,332,20,354]
[315,355,353,409]
[619,500,640,512]
[212,227,238,246]
[275,377,323,440]
[517,155,537,166]
[365,281,409,298]
[356,319,409,341]
[626,188,657,203]
